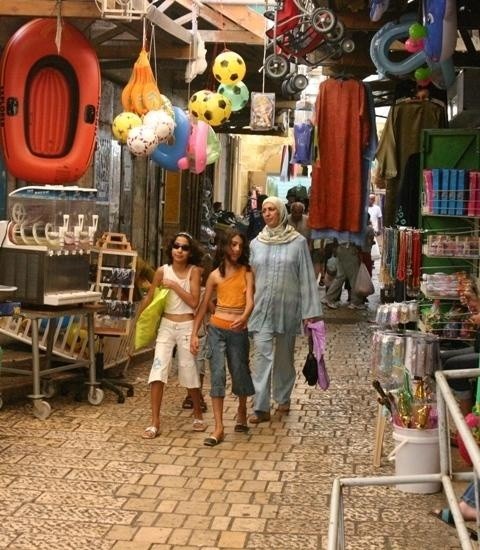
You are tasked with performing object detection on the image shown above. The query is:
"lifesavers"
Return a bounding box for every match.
[186,118,207,174]
[149,106,191,171]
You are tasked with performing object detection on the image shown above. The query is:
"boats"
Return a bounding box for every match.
[0,17,102,183]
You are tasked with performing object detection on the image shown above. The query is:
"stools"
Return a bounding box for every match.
[72,327,135,403]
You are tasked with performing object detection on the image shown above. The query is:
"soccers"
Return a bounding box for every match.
[142,110,175,143]
[127,126,159,158]
[213,50,247,84]
[112,111,142,143]
[201,93,232,126]
[188,90,212,119]
[217,81,249,112]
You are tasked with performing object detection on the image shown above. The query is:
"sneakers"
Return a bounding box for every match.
[348,303,367,309]
[320,298,339,309]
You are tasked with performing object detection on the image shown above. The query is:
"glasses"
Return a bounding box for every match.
[172,241,190,251]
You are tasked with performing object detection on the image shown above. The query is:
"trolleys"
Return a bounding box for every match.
[0,303,107,420]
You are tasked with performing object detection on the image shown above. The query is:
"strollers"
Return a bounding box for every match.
[279,32,355,95]
[264,1,345,78]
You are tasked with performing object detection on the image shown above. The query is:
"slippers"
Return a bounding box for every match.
[430,508,478,541]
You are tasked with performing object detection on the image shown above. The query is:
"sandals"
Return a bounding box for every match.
[142,395,290,446]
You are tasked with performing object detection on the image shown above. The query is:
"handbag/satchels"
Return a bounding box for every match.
[302,353,317,386]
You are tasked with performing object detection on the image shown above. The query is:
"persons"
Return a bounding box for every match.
[209,200,225,225]
[248,195,324,422]
[189,230,255,445]
[310,214,372,309]
[366,192,383,235]
[287,201,312,237]
[456,477,480,525]
[137,232,209,439]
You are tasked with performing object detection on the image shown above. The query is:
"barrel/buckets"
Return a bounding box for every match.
[390,422,440,494]
